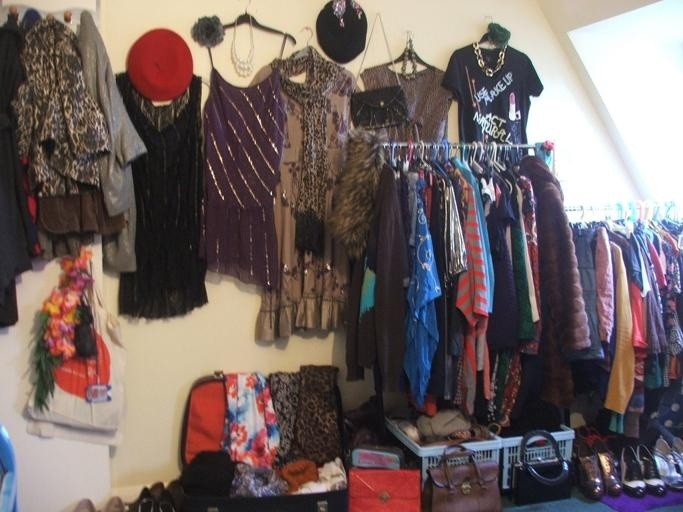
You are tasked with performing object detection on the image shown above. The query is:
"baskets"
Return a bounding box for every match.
[383,416,575,490]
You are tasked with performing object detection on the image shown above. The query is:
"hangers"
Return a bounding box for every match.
[363,29,446,74]
[273,25,326,67]
[380,136,534,181]
[199,0,298,46]
[567,196,680,225]
[470,11,510,52]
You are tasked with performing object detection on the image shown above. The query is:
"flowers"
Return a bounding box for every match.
[540,139,553,161]
[18,237,97,416]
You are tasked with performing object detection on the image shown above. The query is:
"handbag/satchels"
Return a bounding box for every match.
[27,304,125,432]
[348,470,422,511]
[352,443,403,470]
[510,430,572,507]
[350,87,410,130]
[423,445,502,512]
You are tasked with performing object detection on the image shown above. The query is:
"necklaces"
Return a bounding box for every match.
[402,38,417,82]
[472,41,506,80]
[233,15,256,81]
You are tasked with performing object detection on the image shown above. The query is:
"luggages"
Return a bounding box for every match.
[179,370,347,512]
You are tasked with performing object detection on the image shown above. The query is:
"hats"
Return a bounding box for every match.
[316,1,368,64]
[126,29,194,100]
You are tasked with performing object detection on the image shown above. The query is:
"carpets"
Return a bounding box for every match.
[597,484,682,512]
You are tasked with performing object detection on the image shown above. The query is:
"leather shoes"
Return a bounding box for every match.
[578,436,682,501]
[74,481,176,512]
